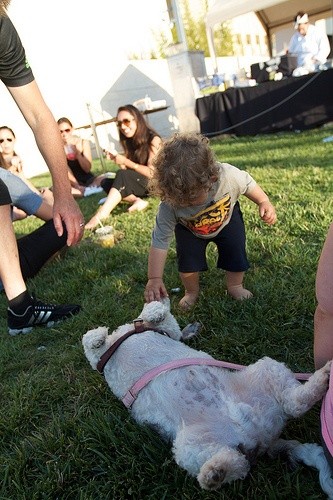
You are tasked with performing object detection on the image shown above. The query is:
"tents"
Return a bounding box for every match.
[97,53,268,155]
[206,0,333,80]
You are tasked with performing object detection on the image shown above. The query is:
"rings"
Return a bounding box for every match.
[80,223,84,226]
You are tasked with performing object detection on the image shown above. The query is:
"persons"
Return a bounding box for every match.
[287,11,330,71]
[143,131,278,312]
[83,104,164,231]
[0,1,86,335]
[48,117,117,200]
[313,221,333,459]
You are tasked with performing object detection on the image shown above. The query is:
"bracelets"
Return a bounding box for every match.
[133,163,137,170]
[148,277,162,280]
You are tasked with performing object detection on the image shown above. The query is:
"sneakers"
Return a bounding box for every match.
[6,291,83,336]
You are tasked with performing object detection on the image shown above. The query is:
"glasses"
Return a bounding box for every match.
[0,137,12,143]
[60,129,70,133]
[117,118,135,128]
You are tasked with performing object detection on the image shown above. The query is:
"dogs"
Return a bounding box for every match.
[79,299,333,494]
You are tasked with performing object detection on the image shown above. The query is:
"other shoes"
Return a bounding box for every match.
[83,184,103,196]
